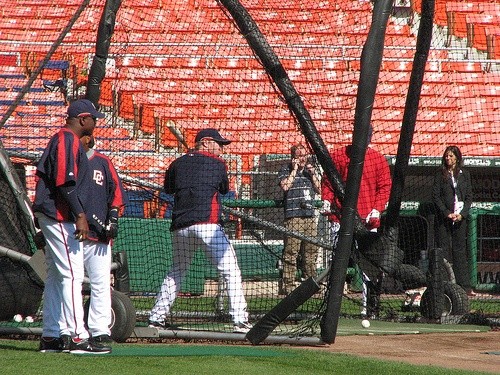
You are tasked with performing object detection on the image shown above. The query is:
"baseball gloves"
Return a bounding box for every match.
[33,216,46,251]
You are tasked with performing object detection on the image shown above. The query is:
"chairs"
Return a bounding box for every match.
[0,0,500,218]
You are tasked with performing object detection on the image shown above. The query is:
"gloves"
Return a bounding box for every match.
[106,216,119,239]
[331,222,340,233]
[366,209,380,230]
[320,200,331,215]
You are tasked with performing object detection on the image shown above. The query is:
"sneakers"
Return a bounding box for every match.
[149,318,176,331]
[232,320,259,334]
[40,335,112,355]
[282,282,300,295]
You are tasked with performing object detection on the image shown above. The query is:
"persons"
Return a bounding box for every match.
[32,100,113,355]
[278,145,322,299]
[321,124,391,318]
[60,131,127,349]
[148,128,253,334]
[432,146,477,296]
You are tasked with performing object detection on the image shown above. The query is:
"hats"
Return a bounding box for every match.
[68,99,104,119]
[194,128,232,145]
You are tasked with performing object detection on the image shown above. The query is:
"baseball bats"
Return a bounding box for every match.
[166,120,191,152]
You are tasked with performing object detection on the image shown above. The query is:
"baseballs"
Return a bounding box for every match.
[361,320,371,328]
